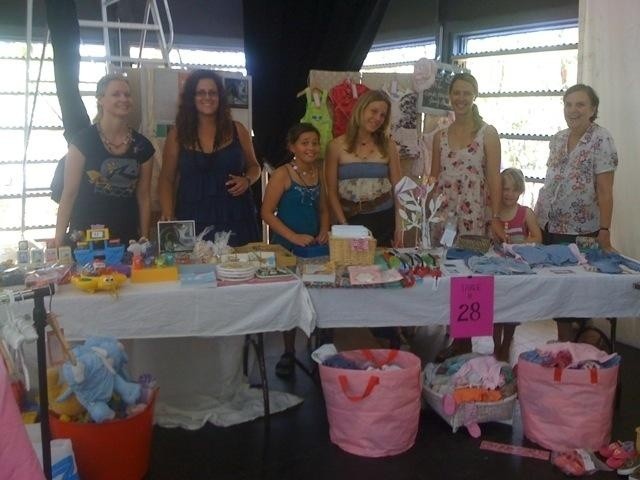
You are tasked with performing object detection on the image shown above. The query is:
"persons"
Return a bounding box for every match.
[490,168,543,362]
[259,123,330,376]
[325,90,405,249]
[425,73,510,362]
[158,69,262,248]
[533,84,618,344]
[55,73,155,253]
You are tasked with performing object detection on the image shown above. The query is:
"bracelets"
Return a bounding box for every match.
[493,213,501,218]
[339,220,348,225]
[245,175,251,187]
[599,227,609,230]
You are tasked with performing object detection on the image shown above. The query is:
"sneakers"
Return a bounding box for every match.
[554,440,640,480]
[276,358,295,378]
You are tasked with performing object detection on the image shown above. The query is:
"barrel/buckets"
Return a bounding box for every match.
[48,387,161,479]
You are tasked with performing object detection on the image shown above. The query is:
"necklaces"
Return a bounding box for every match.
[289,159,320,189]
[98,123,133,148]
[353,136,376,160]
[196,134,218,163]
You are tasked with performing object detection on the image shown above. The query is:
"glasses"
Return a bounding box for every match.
[199,89,219,99]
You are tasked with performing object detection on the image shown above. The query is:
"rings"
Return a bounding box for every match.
[505,235,508,237]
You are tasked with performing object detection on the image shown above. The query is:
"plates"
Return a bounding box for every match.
[217,262,255,282]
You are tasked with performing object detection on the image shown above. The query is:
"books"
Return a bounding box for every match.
[296,258,336,282]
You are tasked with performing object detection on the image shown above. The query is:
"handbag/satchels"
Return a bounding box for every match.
[319,348,422,458]
[517,341,620,453]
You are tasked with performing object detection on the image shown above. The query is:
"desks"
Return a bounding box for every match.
[0,242,304,436]
[298,243,640,436]
